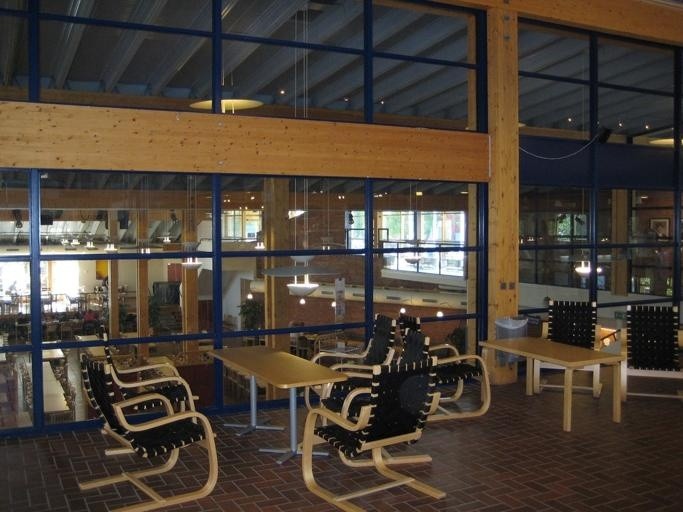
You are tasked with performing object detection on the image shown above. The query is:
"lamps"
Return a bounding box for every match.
[189,0,264,115]
[11,172,204,271]
[261,1,343,304]
[572,26,604,276]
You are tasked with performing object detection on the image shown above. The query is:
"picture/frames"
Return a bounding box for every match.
[648,218,671,241]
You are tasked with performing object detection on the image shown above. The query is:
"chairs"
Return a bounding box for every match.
[302,313,492,512]
[622,303,682,403]
[73,331,157,360]
[80,351,218,511]
[0,309,137,341]
[5,339,77,424]
[532,298,599,399]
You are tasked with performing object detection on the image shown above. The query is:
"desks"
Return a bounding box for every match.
[205,345,348,465]
[478,335,624,433]
